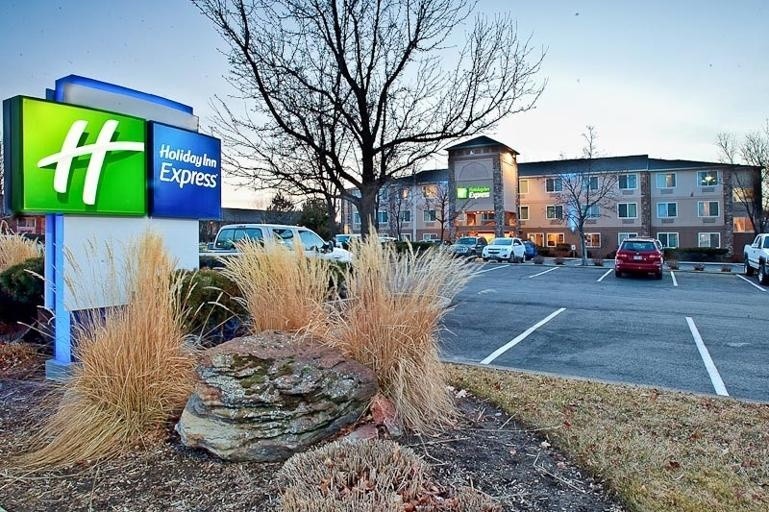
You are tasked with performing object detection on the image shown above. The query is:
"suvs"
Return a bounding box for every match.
[448,236,487,262]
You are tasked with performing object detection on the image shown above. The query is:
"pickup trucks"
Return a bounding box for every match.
[744,234,769,284]
[198,223,355,263]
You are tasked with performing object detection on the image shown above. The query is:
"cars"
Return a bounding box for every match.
[482,237,538,263]
[614,238,663,277]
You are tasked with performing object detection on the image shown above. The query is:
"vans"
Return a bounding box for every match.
[335,233,361,246]
